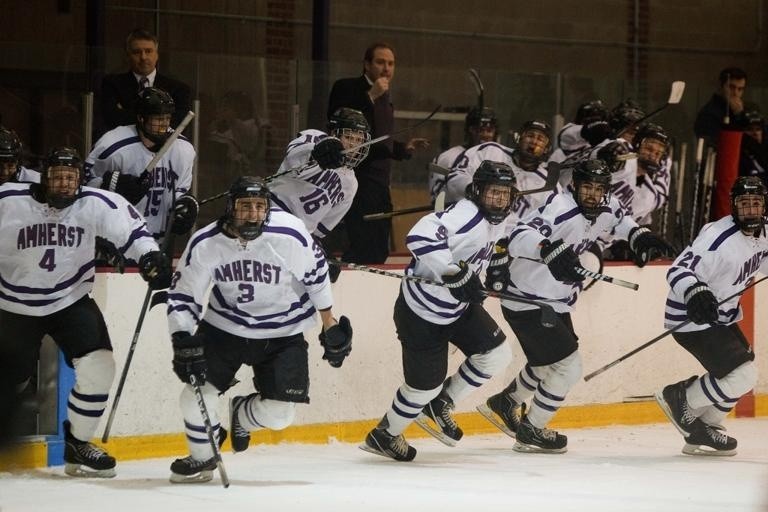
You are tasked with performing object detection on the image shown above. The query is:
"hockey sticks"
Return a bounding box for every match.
[150,291,230,488]
[424,152,643,176]
[197,105,441,206]
[328,260,557,328]
[469,66,484,147]
[435,190,640,290]
[362,161,561,220]
[557,81,685,163]
[101,194,200,443]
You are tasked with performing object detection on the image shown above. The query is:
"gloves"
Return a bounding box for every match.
[683,283,718,324]
[311,136,346,169]
[174,193,199,235]
[143,250,174,289]
[447,229,653,303]
[102,169,147,205]
[320,315,352,369]
[170,336,206,381]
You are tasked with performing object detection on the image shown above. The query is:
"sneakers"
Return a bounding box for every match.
[361,382,570,460]
[231,396,249,452]
[654,382,741,457]
[58,422,117,471]
[171,457,222,473]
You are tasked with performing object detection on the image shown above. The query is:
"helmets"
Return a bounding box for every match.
[41,149,83,198]
[0,128,23,163]
[226,176,271,240]
[729,176,767,231]
[137,88,174,151]
[465,99,669,214]
[741,112,765,130]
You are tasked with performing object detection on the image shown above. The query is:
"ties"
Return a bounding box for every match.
[138,78,148,97]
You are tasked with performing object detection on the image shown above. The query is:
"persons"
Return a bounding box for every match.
[187,90,246,217]
[548,99,617,174]
[583,123,673,246]
[427,101,506,202]
[657,176,768,455]
[445,118,555,213]
[326,40,431,260]
[692,61,768,225]
[227,88,286,175]
[266,106,370,290]
[3,124,43,188]
[557,98,649,160]
[170,174,355,485]
[83,86,198,258]
[0,141,173,479]
[357,159,515,462]
[92,30,195,143]
[478,156,677,456]
